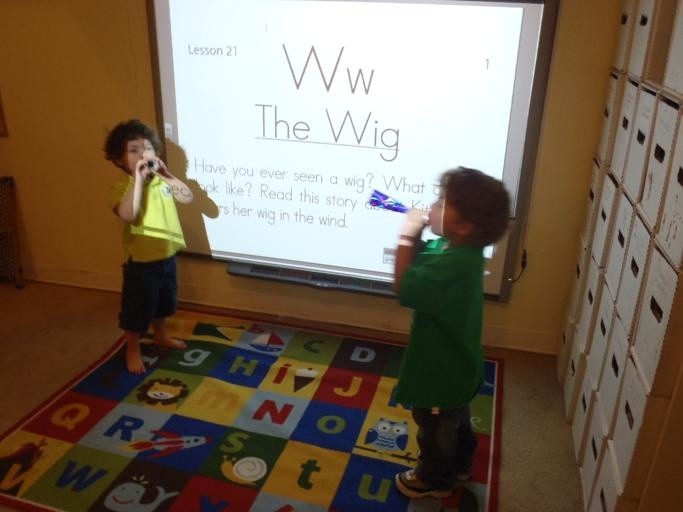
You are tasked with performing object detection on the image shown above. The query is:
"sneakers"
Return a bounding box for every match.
[394,468,457,498]
[416,449,472,480]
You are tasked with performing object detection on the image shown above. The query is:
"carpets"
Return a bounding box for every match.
[0,306,506,512]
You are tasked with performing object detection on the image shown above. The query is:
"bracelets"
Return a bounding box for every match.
[397,239,413,248]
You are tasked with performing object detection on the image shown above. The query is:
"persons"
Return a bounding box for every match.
[392,166,511,500]
[103,119,195,375]
